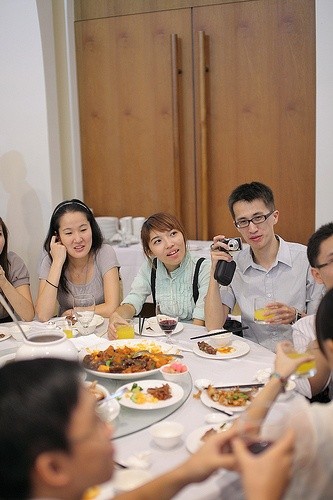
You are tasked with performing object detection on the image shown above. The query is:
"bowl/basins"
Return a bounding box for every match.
[158,363,189,383]
[11,324,29,341]
[110,470,148,493]
[152,423,182,448]
[208,329,233,347]
[84,382,108,409]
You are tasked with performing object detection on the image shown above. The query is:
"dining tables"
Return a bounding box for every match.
[0,317,295,500]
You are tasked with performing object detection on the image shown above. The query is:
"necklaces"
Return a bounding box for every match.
[70,262,87,278]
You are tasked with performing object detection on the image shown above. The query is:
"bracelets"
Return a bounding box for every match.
[270,372,286,393]
[46,280,59,288]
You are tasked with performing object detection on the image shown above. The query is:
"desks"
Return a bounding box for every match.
[52,237,251,317]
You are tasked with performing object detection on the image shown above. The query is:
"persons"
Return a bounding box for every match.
[0,217,36,323]
[0,354,297,500]
[107,212,222,342]
[34,199,121,323]
[224,285,333,500]
[204,181,326,354]
[290,221,333,400]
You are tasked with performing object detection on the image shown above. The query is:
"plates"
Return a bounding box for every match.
[186,425,226,454]
[132,320,185,338]
[201,385,265,412]
[205,413,228,425]
[116,379,185,410]
[76,313,105,326]
[79,345,176,379]
[101,398,121,421]
[95,216,118,243]
[0,327,12,341]
[193,338,250,359]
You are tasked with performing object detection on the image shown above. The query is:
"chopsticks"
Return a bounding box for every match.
[191,325,249,339]
[204,384,263,389]
[138,315,146,333]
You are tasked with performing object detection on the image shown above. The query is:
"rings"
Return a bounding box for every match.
[209,244,215,251]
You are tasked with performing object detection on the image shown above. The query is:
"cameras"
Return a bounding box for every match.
[219,237,243,252]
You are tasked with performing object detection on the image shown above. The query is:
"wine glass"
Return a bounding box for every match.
[74,294,96,336]
[156,301,179,344]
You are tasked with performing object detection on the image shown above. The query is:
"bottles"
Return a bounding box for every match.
[16,330,79,362]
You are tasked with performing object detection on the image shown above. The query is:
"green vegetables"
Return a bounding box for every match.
[225,390,249,400]
[105,355,115,367]
[131,383,143,392]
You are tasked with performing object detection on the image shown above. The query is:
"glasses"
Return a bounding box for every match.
[234,209,278,228]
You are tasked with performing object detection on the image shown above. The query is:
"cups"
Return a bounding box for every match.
[254,295,277,327]
[243,427,274,456]
[120,216,145,237]
[283,344,317,378]
[64,328,73,340]
[114,319,134,340]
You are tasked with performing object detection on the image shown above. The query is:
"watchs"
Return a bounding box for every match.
[290,307,302,325]
